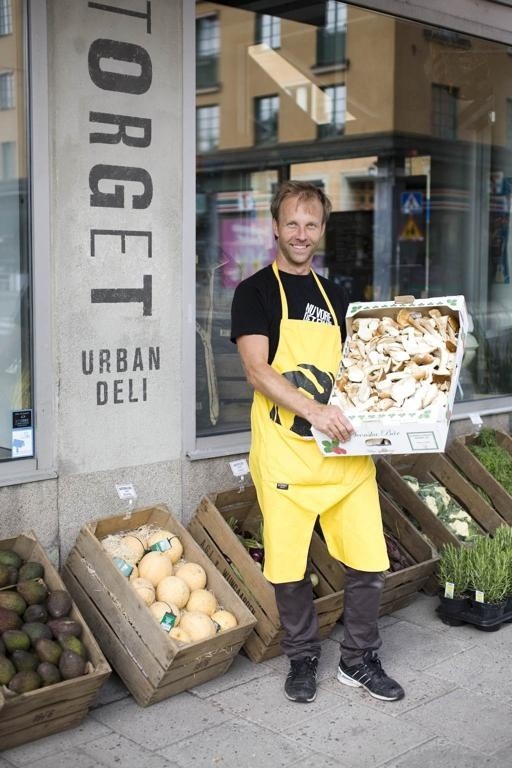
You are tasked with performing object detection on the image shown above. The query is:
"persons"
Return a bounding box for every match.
[231,180,403,702]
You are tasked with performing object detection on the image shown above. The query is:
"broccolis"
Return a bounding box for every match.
[403,474,474,543]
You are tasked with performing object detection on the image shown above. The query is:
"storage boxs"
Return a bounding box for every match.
[311,295,470,459]
[185,484,346,664]
[0,530,111,751]
[343,422,512,634]
[58,501,258,708]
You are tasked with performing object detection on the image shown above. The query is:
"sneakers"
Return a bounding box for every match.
[336,648,405,702]
[284,655,319,703]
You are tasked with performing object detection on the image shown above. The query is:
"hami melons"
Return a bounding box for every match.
[100,526,238,648]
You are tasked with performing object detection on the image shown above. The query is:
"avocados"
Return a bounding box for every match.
[0,549,84,699]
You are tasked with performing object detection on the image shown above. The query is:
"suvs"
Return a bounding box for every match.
[322,205,426,297]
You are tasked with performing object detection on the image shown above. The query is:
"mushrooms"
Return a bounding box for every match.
[331,307,460,415]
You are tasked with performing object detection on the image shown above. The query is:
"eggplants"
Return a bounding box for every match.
[225,530,318,603]
[384,536,409,574]
